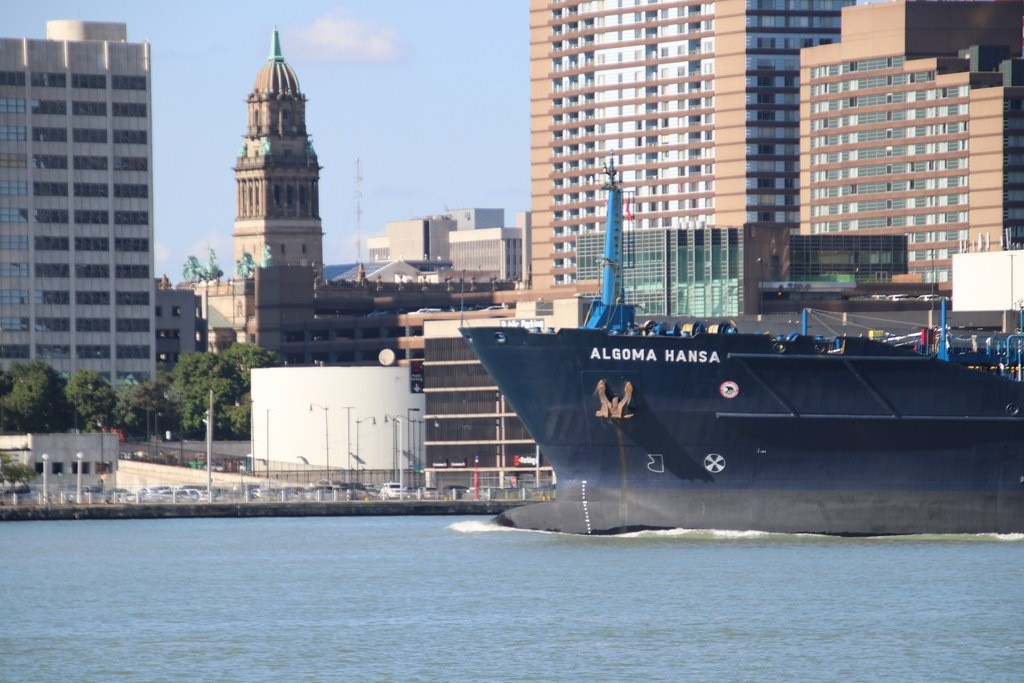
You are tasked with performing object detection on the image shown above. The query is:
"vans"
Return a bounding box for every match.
[111,484,206,502]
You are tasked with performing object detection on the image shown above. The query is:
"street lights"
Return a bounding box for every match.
[384,407,440,491]
[356,417,377,484]
[41,453,50,503]
[309,403,329,485]
[76,453,84,503]
[342,406,356,484]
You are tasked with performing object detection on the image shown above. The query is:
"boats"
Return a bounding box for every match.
[460,148,1024,536]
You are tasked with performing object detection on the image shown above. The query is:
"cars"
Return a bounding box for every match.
[252,481,544,500]
